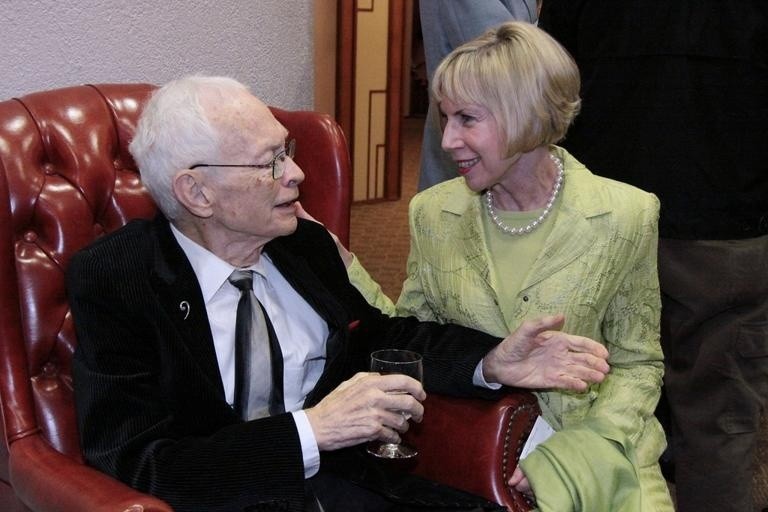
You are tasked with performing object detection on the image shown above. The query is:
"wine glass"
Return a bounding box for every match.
[367,349,423,459]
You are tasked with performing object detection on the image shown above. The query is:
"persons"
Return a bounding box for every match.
[294,22,675,512]
[61,77,609,511]
[420,0,541,190]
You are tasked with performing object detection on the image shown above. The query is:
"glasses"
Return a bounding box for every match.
[189,139,295,178]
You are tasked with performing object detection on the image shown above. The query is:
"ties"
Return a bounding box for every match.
[227,271,285,422]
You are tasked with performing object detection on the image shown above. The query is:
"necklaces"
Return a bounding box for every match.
[485,156,566,235]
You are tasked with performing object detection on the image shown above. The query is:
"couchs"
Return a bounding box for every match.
[0,81,548,510]
